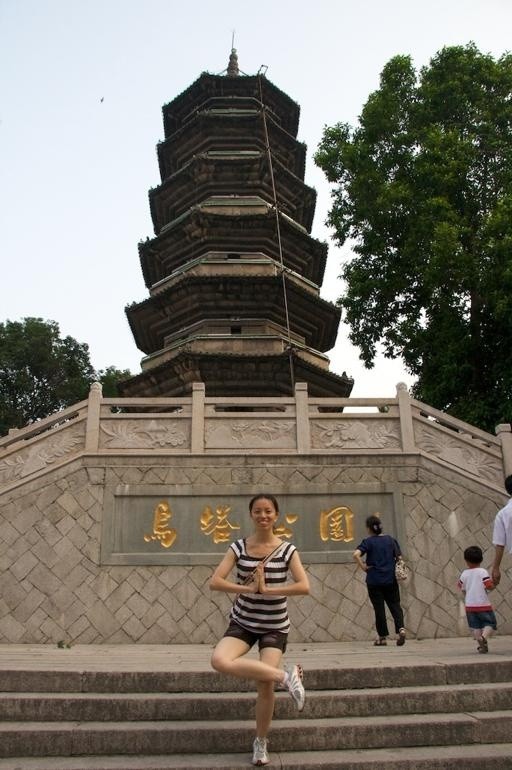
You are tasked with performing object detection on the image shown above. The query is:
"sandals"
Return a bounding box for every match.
[373,637,387,646]
[396,629,406,646]
[476,635,489,654]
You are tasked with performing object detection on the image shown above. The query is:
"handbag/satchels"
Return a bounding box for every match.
[394,559,409,581]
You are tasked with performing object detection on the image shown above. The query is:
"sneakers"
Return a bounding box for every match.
[251,736,270,765]
[282,663,306,712]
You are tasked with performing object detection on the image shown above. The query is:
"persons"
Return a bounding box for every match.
[353,516,406,646]
[456,474,512,653]
[209,494,310,766]
